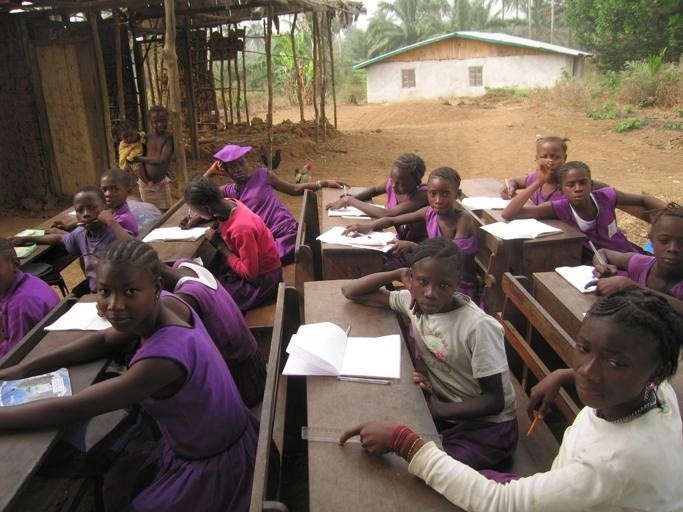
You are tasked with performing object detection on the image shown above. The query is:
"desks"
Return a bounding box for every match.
[301,278,461,511]
[320,187,384,281]
[460,177,587,279]
[12,205,84,298]
[143,204,217,265]
[533,264,681,416]
[0,293,161,510]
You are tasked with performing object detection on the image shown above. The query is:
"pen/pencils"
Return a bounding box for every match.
[505,179,510,194]
[187,208,191,222]
[346,326,351,336]
[76,220,98,225]
[344,185,346,208]
[527,415,538,436]
[1,388,16,402]
[589,241,606,268]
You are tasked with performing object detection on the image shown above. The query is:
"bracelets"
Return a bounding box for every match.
[390,423,421,463]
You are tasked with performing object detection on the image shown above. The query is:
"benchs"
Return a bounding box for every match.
[277,189,316,316]
[1,292,78,369]
[590,180,660,255]
[135,195,185,241]
[452,198,502,291]
[247,284,290,512]
[494,271,582,478]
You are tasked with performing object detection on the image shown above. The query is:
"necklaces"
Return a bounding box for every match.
[608,391,656,425]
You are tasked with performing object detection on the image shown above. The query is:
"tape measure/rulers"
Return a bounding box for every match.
[301,427,443,448]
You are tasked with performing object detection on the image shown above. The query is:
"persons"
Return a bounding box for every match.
[341,238,519,468]
[343,167,478,300]
[326,153,429,271]
[584,201,683,317]
[339,287,683,512]
[501,136,569,206]
[501,160,670,252]
